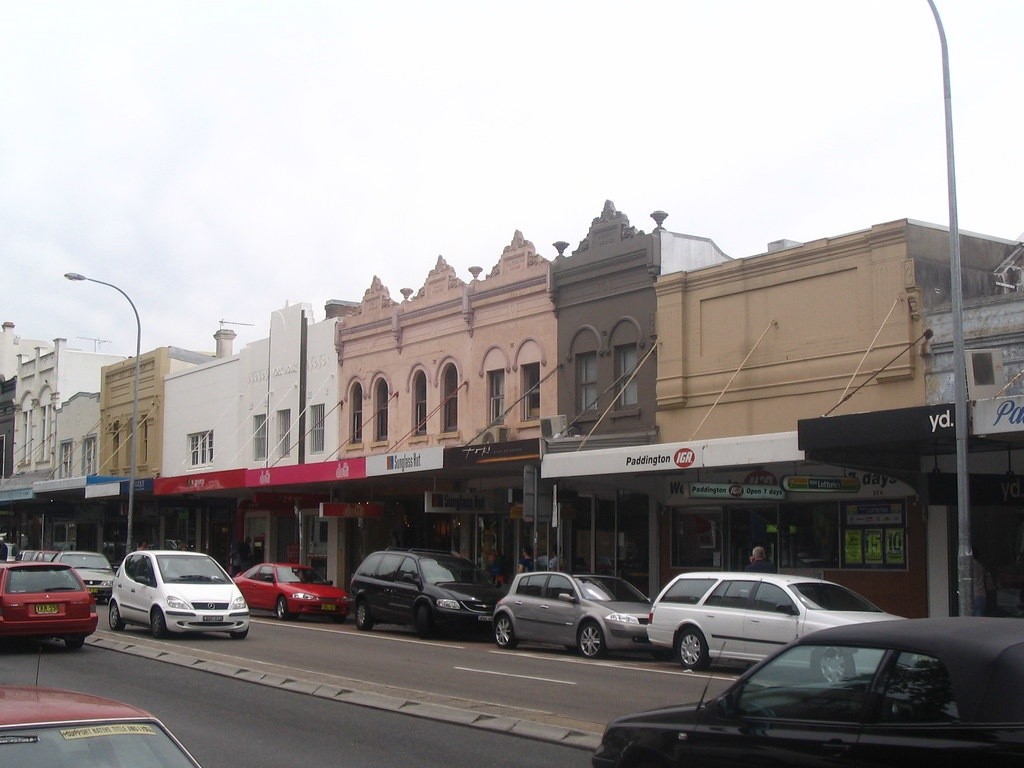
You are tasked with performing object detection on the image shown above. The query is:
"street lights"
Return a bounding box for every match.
[63,272,141,564]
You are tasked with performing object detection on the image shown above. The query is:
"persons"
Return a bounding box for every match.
[226,537,252,576]
[485,554,503,588]
[0,537,34,561]
[516,544,558,572]
[971,545,987,616]
[744,546,775,574]
[133,539,149,551]
[180,544,187,551]
[422,536,458,549]
[1017,521,1024,611]
[387,529,400,548]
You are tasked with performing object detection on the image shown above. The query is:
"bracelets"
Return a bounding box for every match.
[1019,552,1023,555]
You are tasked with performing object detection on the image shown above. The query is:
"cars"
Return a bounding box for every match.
[52,550,117,601]
[0,682,200,768]
[489,569,657,658]
[0,560,99,650]
[16,548,62,565]
[233,562,351,623]
[108,548,249,641]
[593,618,1024,768]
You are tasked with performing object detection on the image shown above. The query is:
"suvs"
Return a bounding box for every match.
[347,548,505,639]
[643,572,939,681]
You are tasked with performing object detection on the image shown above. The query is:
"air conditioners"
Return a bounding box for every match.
[474,426,508,444]
[540,415,567,437]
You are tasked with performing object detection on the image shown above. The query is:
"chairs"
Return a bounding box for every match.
[168,562,181,570]
[881,663,927,723]
